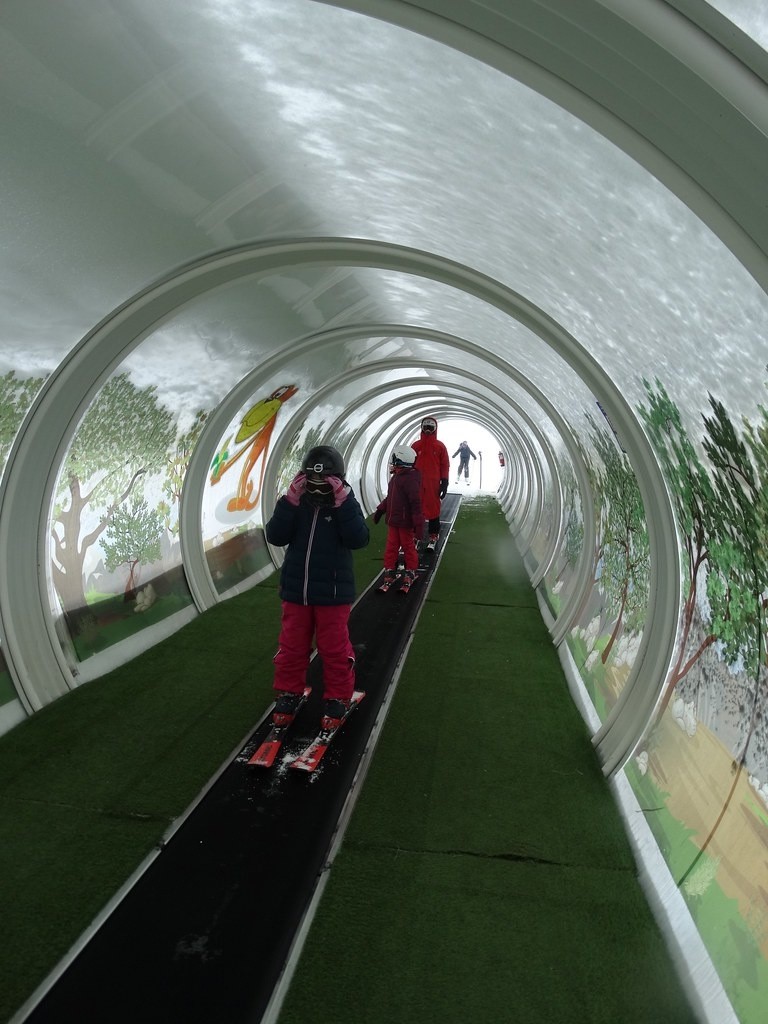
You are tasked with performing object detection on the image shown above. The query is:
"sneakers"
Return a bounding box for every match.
[321,699,350,730]
[383,568,396,583]
[273,690,300,726]
[401,569,415,586]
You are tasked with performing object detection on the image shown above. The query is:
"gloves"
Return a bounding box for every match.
[412,525,424,540]
[373,509,382,524]
[439,479,448,499]
[324,474,351,506]
[286,470,307,506]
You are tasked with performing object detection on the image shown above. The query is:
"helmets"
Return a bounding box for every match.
[302,446,345,479]
[422,419,437,434]
[391,445,415,465]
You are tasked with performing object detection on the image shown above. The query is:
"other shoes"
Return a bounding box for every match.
[429,532,440,542]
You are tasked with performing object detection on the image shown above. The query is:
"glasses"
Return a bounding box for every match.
[305,480,333,495]
[423,425,434,431]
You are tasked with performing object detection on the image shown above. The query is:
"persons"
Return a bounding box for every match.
[265,445,370,733]
[452,441,476,482]
[372,446,426,586]
[410,416,450,541]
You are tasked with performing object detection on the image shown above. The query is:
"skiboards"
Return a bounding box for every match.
[414,532,441,552]
[379,574,419,594]
[246,686,367,773]
[454,479,472,487]
